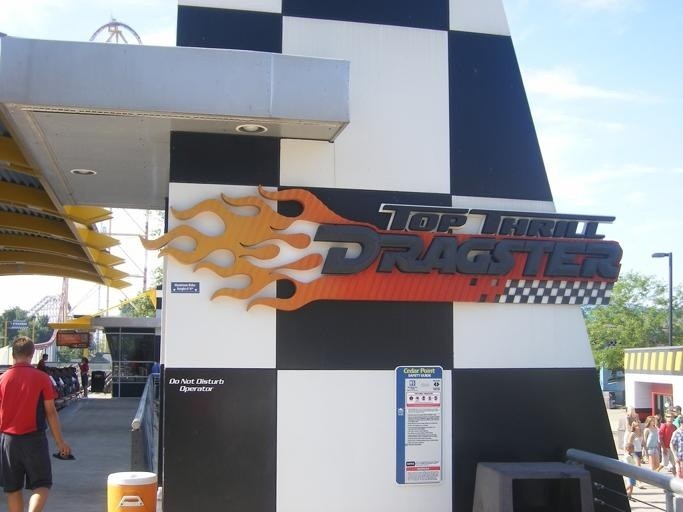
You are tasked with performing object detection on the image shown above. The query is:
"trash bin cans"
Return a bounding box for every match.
[92,371,105,392]
[473,462,594,512]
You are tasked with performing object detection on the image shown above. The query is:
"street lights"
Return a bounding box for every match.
[652,251,674,347]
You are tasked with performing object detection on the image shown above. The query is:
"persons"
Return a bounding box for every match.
[618,404,682,501]
[77,356,89,400]
[37,353,49,369]
[0,337,71,511]
